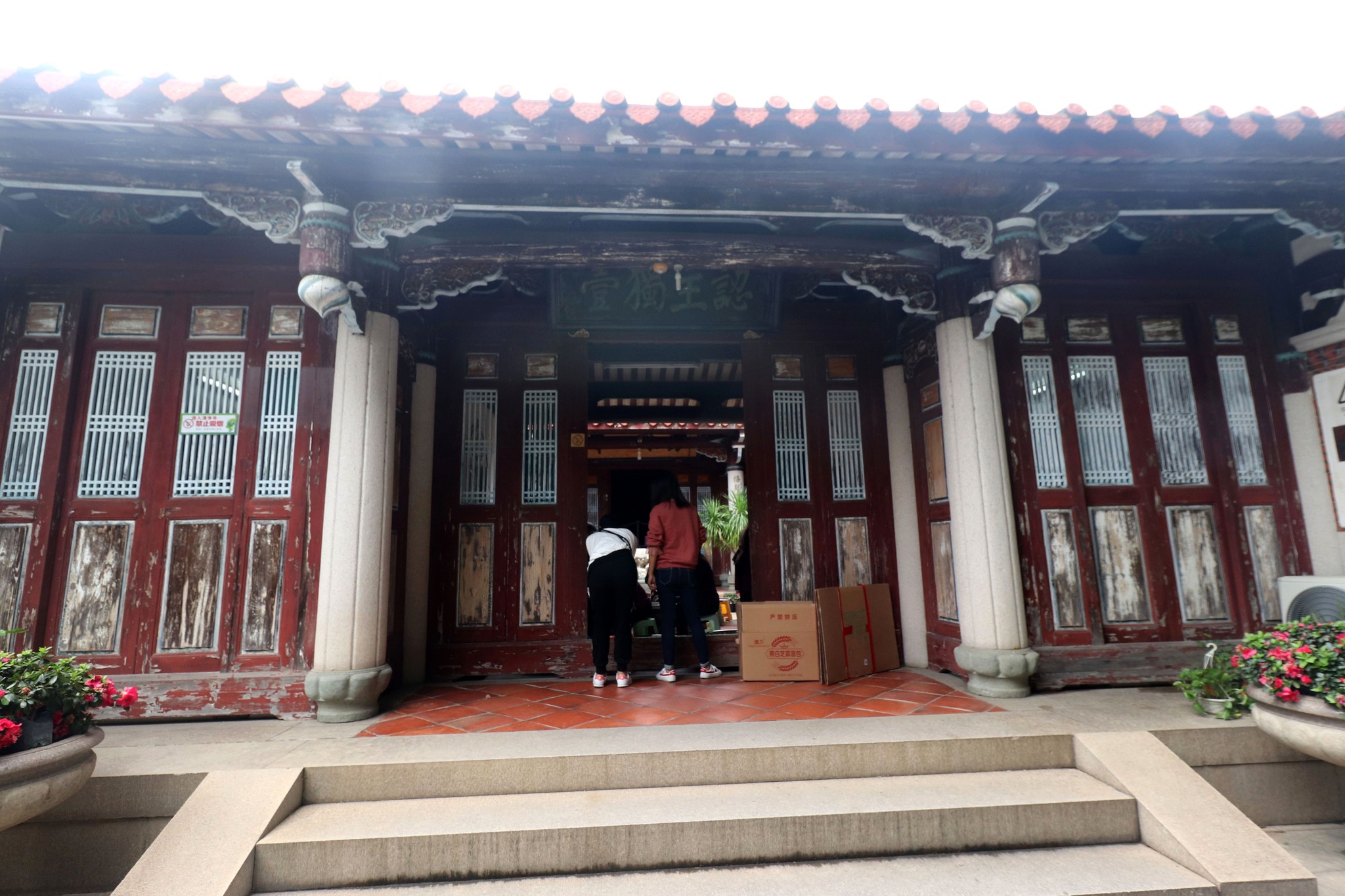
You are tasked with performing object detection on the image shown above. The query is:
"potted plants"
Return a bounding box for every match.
[1172,632,1242,721]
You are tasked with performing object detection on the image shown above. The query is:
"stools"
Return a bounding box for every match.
[700,614,721,633]
[633,617,659,636]
[720,600,733,622]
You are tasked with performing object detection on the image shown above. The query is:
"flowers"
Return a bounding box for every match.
[0,625,141,750]
[1231,614,1345,710]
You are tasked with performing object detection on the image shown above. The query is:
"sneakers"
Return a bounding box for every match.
[656,668,676,682]
[700,664,722,678]
[593,672,607,687]
[616,671,633,687]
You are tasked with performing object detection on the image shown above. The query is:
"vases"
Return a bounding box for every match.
[0,725,106,831]
[1245,681,1345,769]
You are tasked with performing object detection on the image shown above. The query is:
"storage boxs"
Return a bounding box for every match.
[731,596,823,687]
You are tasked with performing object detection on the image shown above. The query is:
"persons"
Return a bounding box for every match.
[645,574,658,604]
[632,580,652,631]
[734,526,755,606]
[638,471,724,681]
[585,513,638,688]
[646,548,720,636]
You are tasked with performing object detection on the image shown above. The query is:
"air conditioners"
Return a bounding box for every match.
[1274,572,1345,625]
